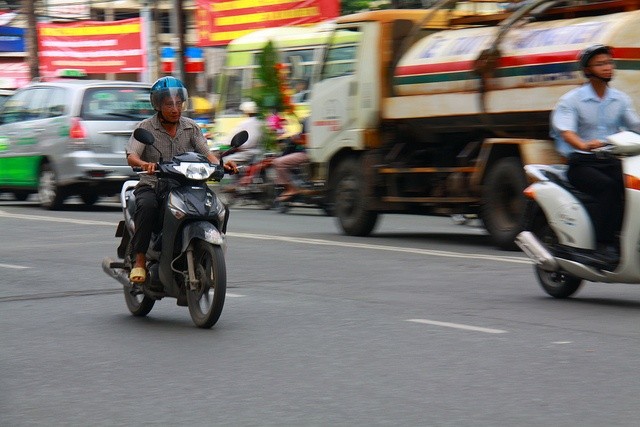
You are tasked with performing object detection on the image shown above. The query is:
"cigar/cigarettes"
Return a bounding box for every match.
[235,164,244,169]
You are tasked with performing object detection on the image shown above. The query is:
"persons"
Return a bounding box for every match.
[125,75,240,283]
[549,44,640,262]
[272,116,309,202]
[204,101,261,167]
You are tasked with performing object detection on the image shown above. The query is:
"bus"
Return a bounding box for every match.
[215,28,362,181]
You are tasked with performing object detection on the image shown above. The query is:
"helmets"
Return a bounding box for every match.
[150,76,189,124]
[578,44,614,83]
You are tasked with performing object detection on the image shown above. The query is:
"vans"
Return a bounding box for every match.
[0,81,156,210]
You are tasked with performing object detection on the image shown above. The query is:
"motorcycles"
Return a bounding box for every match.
[267,142,337,218]
[103,131,247,328]
[514,131,640,298]
[224,152,288,212]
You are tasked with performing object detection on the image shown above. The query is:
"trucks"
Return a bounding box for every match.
[306,2,640,250]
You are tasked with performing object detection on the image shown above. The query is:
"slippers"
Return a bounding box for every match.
[129,264,148,284]
